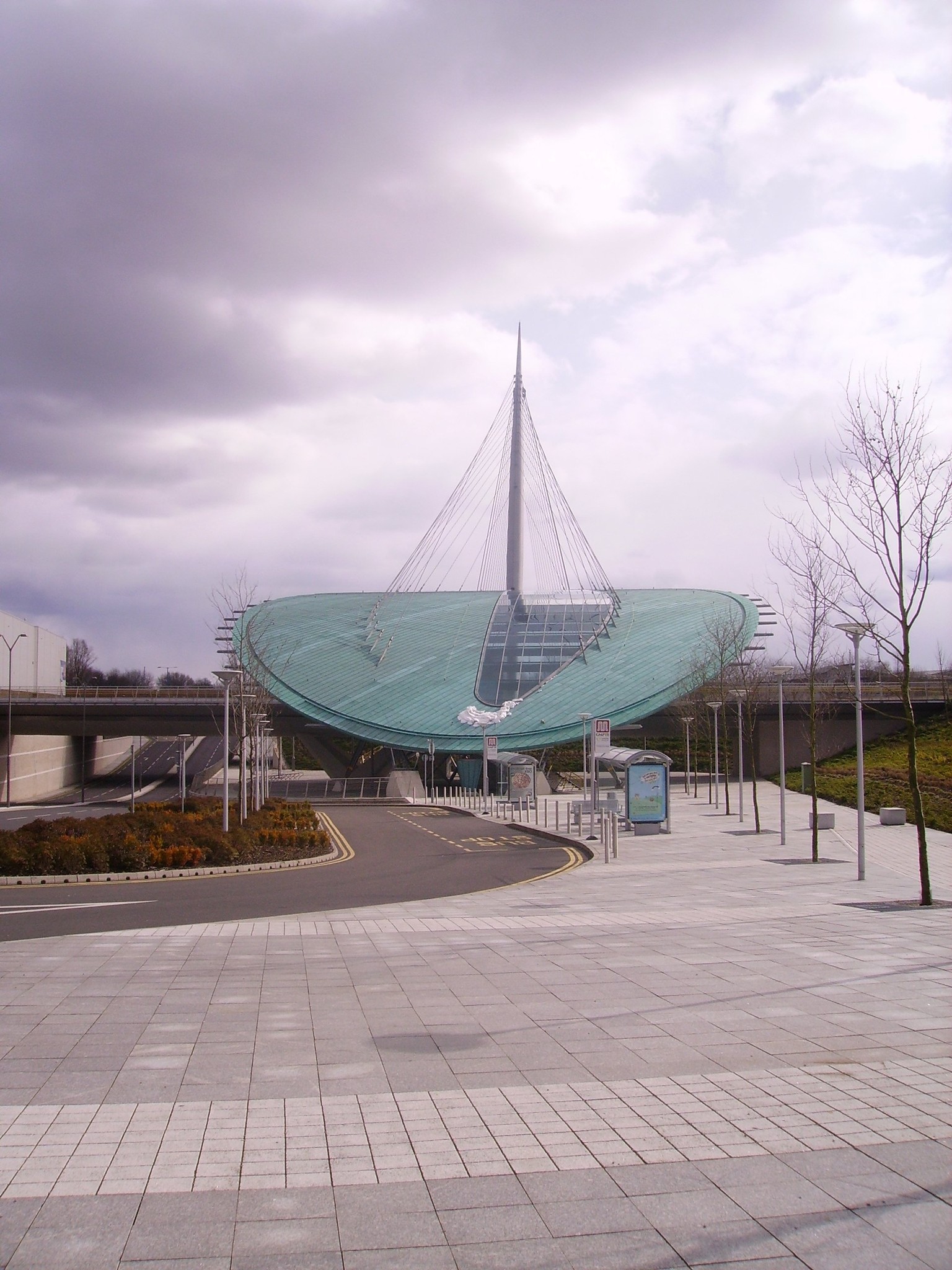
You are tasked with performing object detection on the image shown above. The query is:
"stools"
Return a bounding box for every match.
[879,807,906,825]
[809,812,835,829]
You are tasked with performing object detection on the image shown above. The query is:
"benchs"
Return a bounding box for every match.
[571,799,625,826]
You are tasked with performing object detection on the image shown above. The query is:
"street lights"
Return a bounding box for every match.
[835,623,876,880]
[211,670,243,832]
[233,694,256,820]
[729,690,753,822]
[764,666,795,846]
[681,718,693,795]
[157,666,178,687]
[250,713,274,812]
[578,713,592,802]
[707,702,725,810]
[0,634,28,808]
[73,676,97,802]
[178,734,192,815]
